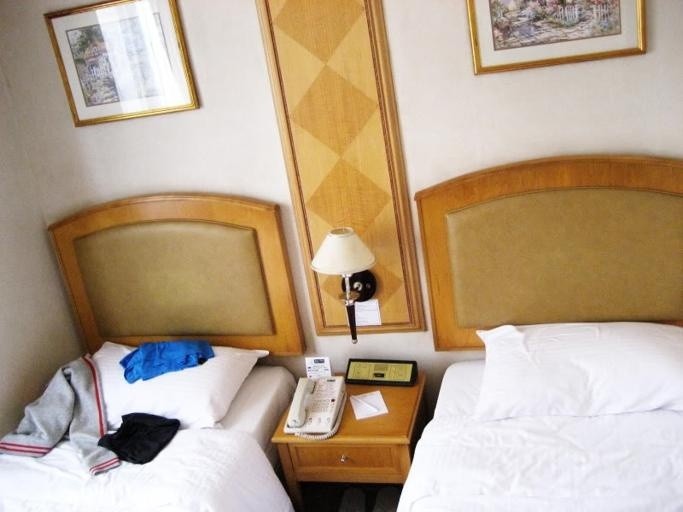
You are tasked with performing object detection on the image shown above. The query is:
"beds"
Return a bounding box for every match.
[0,191,306,512]
[396,153,683,512]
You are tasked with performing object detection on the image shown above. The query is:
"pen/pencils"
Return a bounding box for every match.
[352,396,379,413]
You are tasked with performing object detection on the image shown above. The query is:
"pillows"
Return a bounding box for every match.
[473,321,683,422]
[90,341,270,431]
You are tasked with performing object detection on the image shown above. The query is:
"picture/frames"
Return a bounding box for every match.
[466,0,648,75]
[43,0,200,127]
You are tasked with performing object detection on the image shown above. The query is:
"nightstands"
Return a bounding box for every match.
[270,369,428,512]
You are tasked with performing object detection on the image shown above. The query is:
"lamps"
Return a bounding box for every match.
[309,228,378,345]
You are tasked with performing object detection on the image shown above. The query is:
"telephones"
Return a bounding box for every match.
[283,376,346,434]
